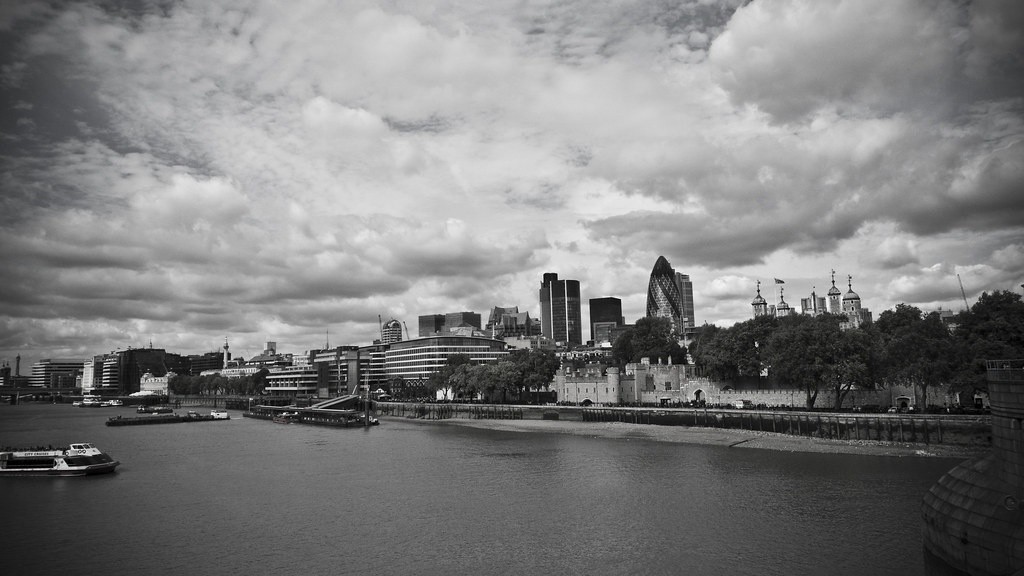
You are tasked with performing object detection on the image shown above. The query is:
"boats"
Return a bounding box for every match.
[272,412,302,425]
[136,405,175,414]
[72,397,124,408]
[241,404,378,428]
[210,410,230,420]
[104,408,215,426]
[0,442,122,478]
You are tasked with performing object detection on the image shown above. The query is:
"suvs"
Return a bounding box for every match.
[736,399,757,410]
